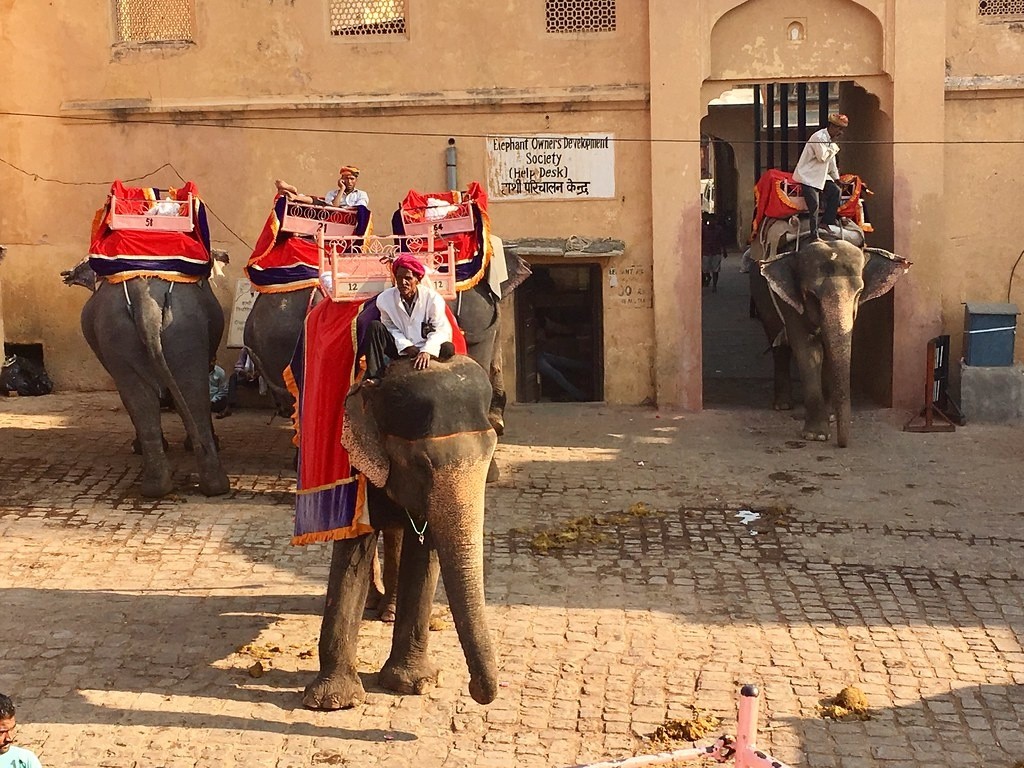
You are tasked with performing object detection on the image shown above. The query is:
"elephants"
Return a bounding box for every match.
[752,198,914,448]
[241,232,534,711]
[79,245,230,499]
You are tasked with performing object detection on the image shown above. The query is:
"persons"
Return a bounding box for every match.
[324,165,371,240]
[361,254,455,390]
[208,345,292,419]
[701,210,728,293]
[792,112,848,244]
[0,694,41,768]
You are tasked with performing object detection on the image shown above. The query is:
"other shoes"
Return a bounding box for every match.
[215,411,232,419]
[818,222,836,234]
[809,238,824,243]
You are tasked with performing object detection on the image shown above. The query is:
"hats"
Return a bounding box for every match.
[392,254,426,281]
[340,166,360,176]
[827,113,848,127]
